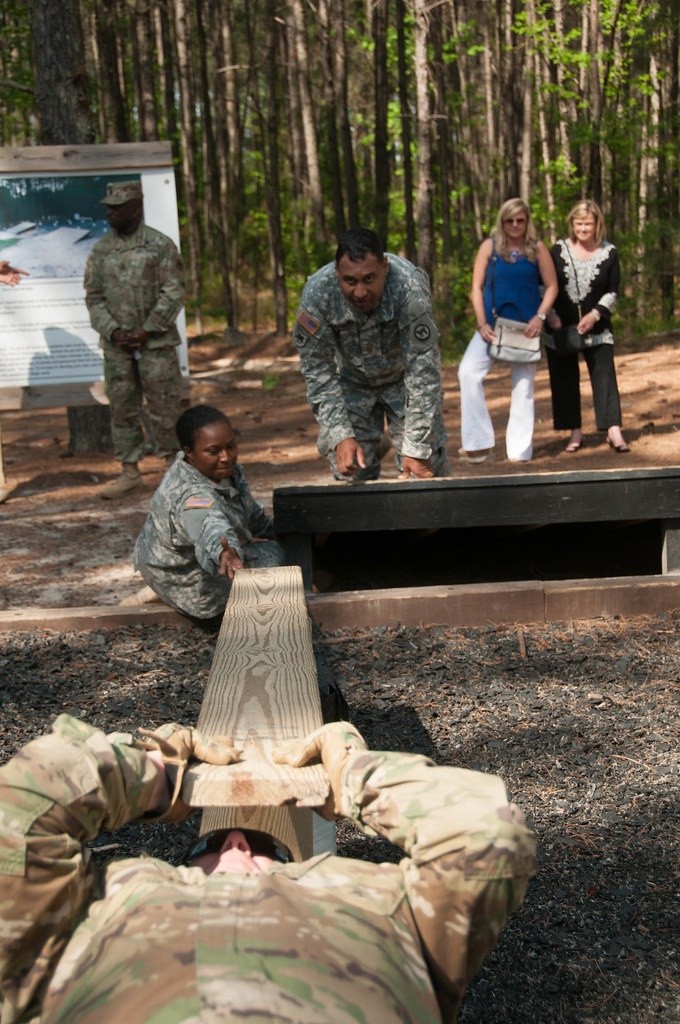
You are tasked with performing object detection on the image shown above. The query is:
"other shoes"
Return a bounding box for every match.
[458,443,492,457]
[100,470,144,499]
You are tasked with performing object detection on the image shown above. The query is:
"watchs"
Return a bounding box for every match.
[537,313,547,321]
[591,311,600,321]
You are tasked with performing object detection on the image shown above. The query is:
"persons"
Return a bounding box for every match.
[83,179,198,500]
[133,405,288,617]
[291,228,452,481]
[458,184,559,467]
[0,715,532,1024]
[541,201,630,454]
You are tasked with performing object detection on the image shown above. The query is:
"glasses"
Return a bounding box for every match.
[504,219,525,224]
[183,828,294,867]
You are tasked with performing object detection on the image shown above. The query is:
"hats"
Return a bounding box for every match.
[100,180,142,205]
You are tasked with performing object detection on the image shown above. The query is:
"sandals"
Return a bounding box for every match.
[564,435,586,452]
[607,436,630,453]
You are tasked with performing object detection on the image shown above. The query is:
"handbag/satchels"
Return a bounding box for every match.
[489,317,541,363]
[567,321,592,347]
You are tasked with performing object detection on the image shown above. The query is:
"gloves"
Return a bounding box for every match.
[130,722,240,823]
[272,721,369,821]
